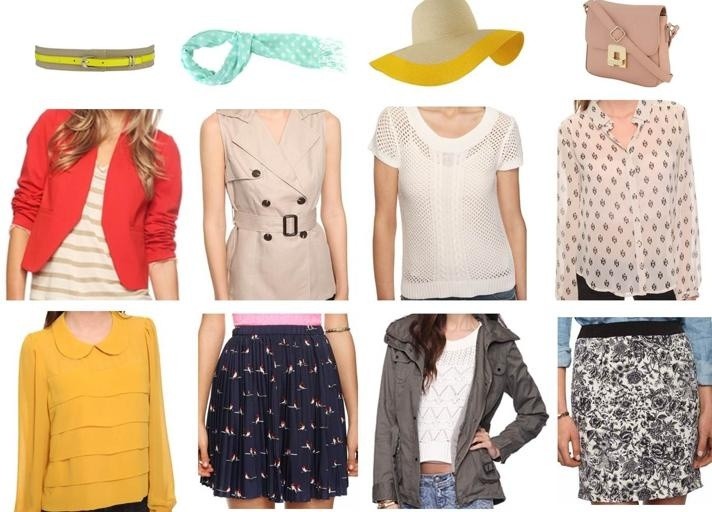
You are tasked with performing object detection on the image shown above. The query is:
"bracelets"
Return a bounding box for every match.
[558,411,569,419]
[377,500,396,508]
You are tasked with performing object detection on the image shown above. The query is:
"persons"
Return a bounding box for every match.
[371,313,550,509]
[554,100,704,301]
[199,109,352,302]
[553,317,712,506]
[371,107,530,300]
[197,313,362,508]
[7,109,181,300]
[14,309,180,511]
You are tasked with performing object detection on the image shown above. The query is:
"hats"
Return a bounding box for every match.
[369,0,525,88]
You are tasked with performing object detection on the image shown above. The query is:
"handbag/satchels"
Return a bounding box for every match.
[582,0,679,88]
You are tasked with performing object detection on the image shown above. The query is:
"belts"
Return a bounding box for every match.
[35,44,156,73]
